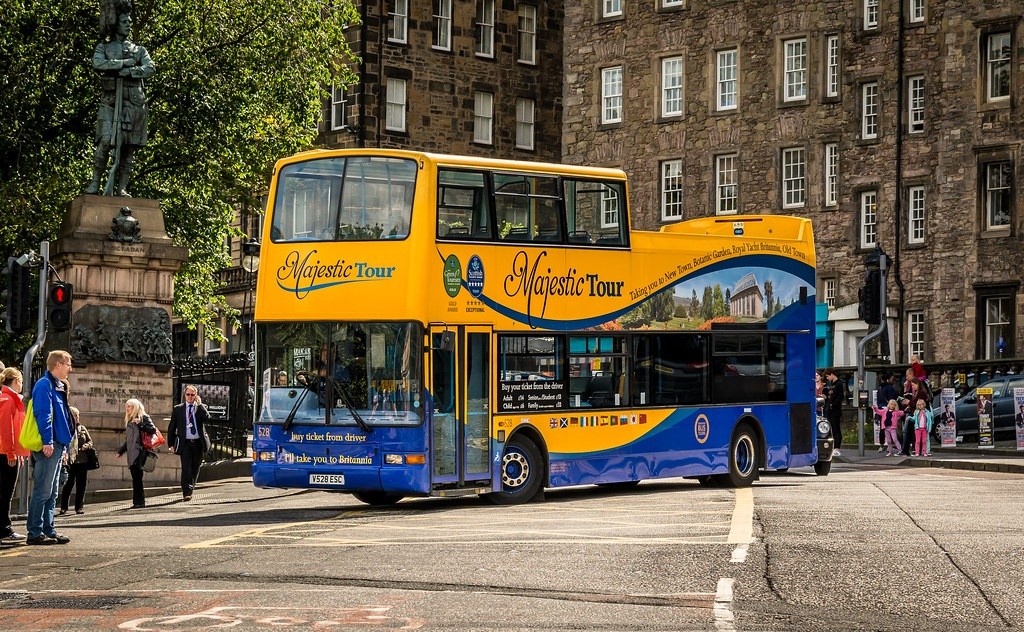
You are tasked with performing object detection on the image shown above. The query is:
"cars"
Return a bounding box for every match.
[777,397,835,476]
[929,371,1024,444]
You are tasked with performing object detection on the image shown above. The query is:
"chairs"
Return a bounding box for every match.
[533,229,558,242]
[480,226,502,239]
[569,231,592,243]
[588,371,614,395]
[505,228,532,240]
[445,227,470,238]
[595,233,620,245]
[586,392,615,407]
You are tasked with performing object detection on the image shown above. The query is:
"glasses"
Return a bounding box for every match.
[186,393,197,397]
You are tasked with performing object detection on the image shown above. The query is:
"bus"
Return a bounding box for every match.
[252,148,819,505]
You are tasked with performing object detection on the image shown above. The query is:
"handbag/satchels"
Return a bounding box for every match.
[139,412,165,449]
[86,447,100,470]
[139,448,159,472]
[18,395,45,453]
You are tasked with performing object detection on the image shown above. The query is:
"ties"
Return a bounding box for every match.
[189,404,197,435]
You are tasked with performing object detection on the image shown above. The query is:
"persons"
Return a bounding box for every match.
[116,398,156,508]
[59,406,93,514]
[907,399,932,456]
[303,343,351,390]
[0,362,30,540]
[26,350,75,545]
[978,394,992,414]
[871,399,905,456]
[942,405,954,425]
[1015,404,1024,428]
[167,385,212,501]
[876,354,943,453]
[815,367,843,449]
[277,371,288,385]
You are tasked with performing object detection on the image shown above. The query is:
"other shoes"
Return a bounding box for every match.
[2,532,26,540]
[132,502,145,509]
[26,534,57,545]
[921,452,927,457]
[886,451,892,456]
[897,449,904,454]
[184,494,191,500]
[76,507,84,514]
[832,450,842,456]
[915,453,919,456]
[879,446,884,451]
[46,530,70,543]
[60,508,67,514]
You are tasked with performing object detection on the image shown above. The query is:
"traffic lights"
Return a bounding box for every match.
[858,268,882,326]
[47,280,74,332]
[0,256,32,334]
